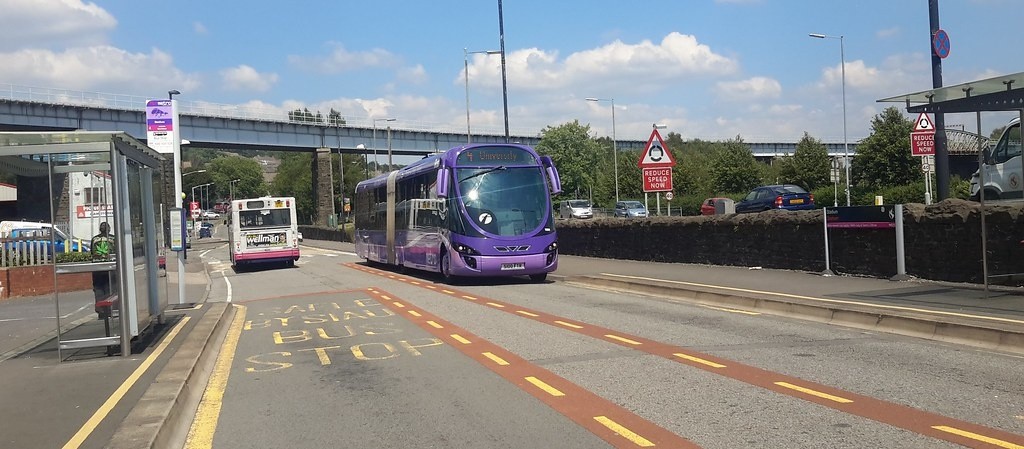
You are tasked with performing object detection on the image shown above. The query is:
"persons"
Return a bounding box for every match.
[91,222,118,319]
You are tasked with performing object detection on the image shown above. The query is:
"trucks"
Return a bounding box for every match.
[559,198,594,220]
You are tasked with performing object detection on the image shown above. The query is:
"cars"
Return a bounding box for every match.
[735,184,815,215]
[4,228,92,263]
[701,198,734,215]
[203,211,220,220]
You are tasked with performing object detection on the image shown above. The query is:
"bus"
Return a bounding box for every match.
[352,143,561,285]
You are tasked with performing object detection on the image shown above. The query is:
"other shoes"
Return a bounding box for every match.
[108,314,111,317]
[99,311,104,320]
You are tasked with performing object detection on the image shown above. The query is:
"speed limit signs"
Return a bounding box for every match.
[665,191,674,200]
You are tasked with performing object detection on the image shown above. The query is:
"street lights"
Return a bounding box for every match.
[809,32,850,207]
[372,117,397,171]
[357,144,369,180]
[464,50,503,143]
[192,184,210,203]
[199,183,215,210]
[586,96,619,202]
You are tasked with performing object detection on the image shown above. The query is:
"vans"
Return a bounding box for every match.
[199,227,212,239]
[223,195,303,273]
[0,220,91,251]
[967,115,1024,201]
[613,200,649,219]
